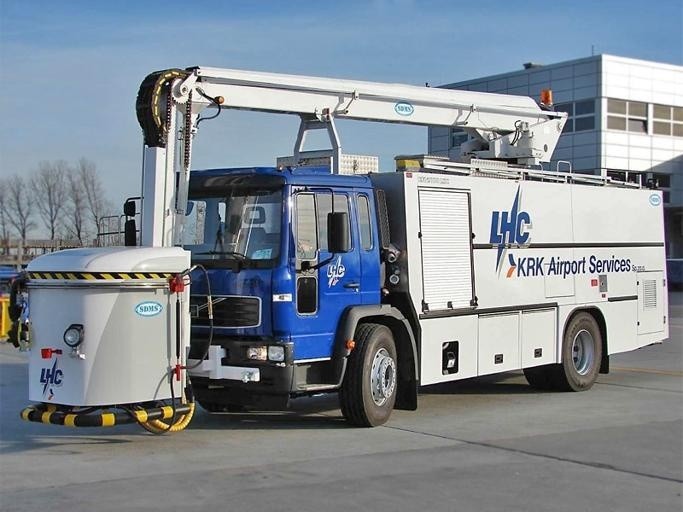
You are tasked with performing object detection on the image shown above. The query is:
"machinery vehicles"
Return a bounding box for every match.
[7,66,670,435]
